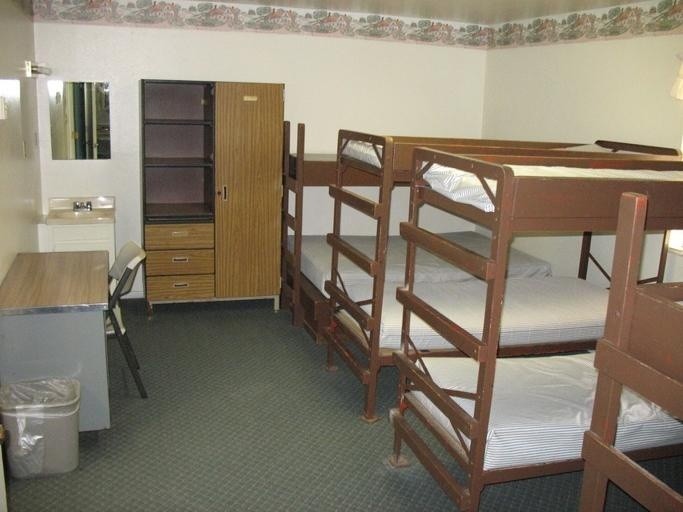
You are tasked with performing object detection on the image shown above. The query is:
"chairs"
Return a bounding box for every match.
[104,239,148,399]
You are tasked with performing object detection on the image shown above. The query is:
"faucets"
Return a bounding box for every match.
[74,201,92,212]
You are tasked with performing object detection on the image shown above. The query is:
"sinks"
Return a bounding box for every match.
[56,210,102,219]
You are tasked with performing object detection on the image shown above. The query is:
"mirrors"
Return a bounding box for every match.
[45,78,110,161]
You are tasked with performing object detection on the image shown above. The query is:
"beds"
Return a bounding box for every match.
[281,121,552,349]
[325,130,678,424]
[577,192,682,510]
[387,147,681,512]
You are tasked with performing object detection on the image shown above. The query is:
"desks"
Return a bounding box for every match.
[0,250,111,433]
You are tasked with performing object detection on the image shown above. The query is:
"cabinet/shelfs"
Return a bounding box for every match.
[138,79,286,322]
[46,225,126,337]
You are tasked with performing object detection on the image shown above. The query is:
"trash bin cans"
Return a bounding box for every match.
[0,378,81,479]
